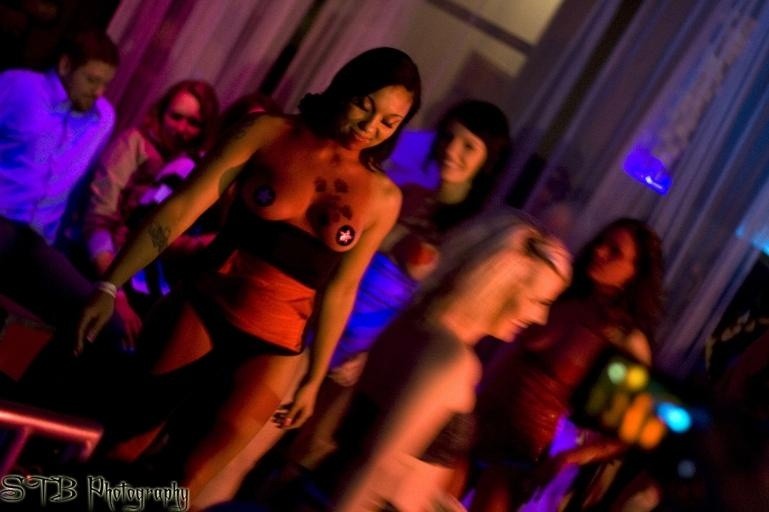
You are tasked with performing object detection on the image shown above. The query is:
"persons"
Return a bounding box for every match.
[0,22,767,512]
